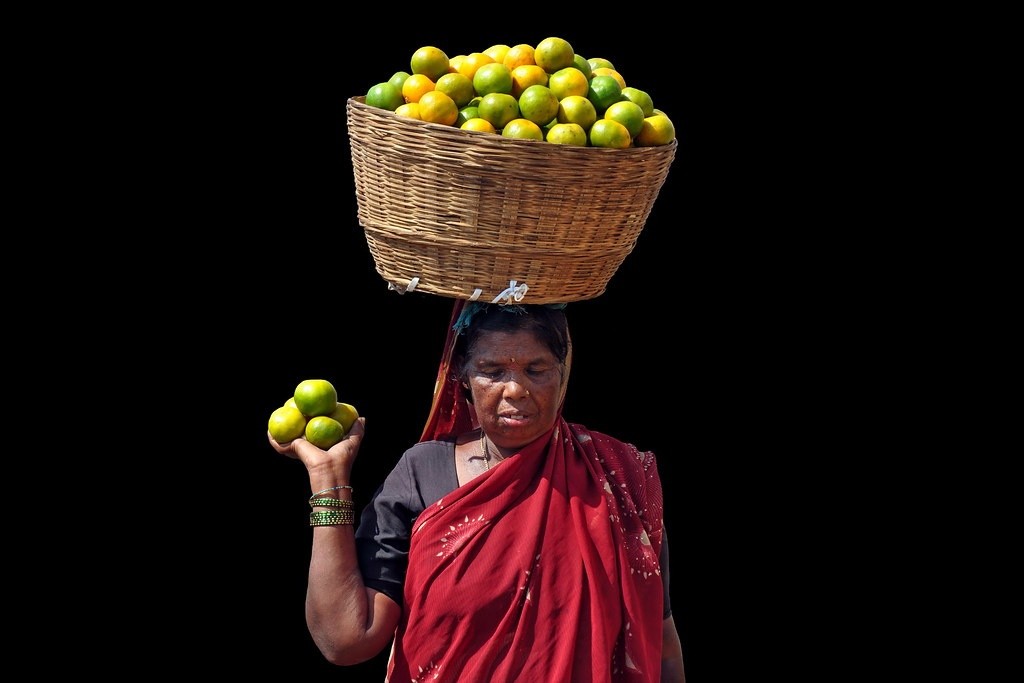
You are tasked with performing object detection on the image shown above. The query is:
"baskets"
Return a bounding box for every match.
[344,91,678,304]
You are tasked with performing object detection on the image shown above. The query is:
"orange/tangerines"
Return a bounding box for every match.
[367,36,675,147]
[269,379,360,449]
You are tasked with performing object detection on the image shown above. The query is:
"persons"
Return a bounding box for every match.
[266,292,686,683]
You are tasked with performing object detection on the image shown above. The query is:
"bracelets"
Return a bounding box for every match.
[308,486,354,526]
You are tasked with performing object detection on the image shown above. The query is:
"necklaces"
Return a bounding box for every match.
[481,430,490,471]
